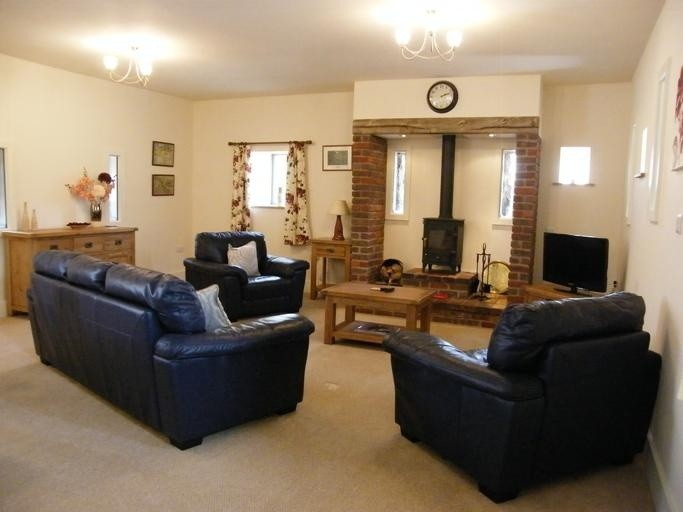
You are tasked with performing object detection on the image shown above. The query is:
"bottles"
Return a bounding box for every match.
[30,209,37,231]
[19,202,28,232]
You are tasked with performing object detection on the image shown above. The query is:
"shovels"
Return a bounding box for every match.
[469,254,479,295]
[481,254,491,293]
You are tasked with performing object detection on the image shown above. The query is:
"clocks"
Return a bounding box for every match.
[426,78,457,113]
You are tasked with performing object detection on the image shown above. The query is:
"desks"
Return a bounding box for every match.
[305,235,351,301]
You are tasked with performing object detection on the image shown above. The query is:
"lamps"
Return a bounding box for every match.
[326,201,351,242]
[387,7,460,64]
[65,29,157,91]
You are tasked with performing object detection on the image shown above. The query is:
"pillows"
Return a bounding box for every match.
[226,241,261,276]
[194,284,236,331]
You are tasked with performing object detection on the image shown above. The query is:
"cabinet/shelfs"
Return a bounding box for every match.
[524,283,600,306]
[1,224,138,317]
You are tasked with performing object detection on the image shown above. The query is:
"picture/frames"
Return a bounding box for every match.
[152,139,175,169]
[152,173,175,196]
[321,144,355,172]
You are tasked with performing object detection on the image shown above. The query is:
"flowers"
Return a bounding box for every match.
[67,164,117,203]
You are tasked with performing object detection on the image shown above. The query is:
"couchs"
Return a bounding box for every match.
[26,245,317,447]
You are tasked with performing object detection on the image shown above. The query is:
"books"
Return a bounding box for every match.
[354,324,400,335]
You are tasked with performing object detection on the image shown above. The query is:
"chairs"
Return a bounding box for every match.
[382,290,662,505]
[182,229,310,320]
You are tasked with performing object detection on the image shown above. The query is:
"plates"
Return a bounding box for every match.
[67,222,90,228]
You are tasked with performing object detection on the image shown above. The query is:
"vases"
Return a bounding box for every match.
[30,209,38,232]
[20,201,28,232]
[90,200,103,220]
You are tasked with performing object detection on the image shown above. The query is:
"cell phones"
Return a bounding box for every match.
[381,287,394,292]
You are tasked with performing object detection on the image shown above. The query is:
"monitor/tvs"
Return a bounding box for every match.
[542,231,609,296]
